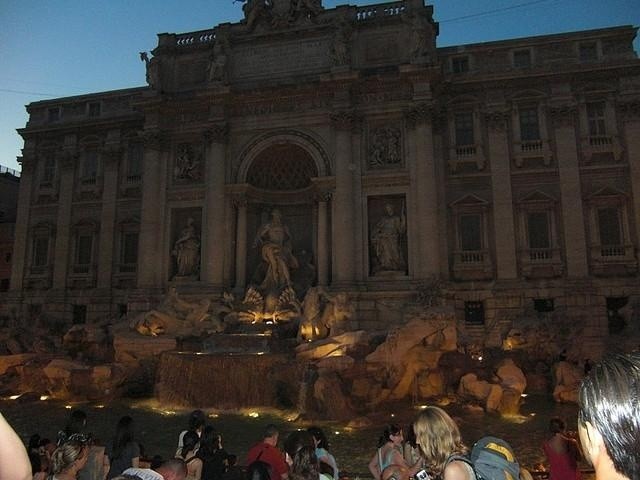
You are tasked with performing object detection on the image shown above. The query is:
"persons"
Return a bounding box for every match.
[25,410,339,480]
[172,214,199,274]
[172,295,212,327]
[543,417,583,480]
[318,288,354,336]
[256,208,299,292]
[368,406,532,480]
[576,350,640,480]
[0,413,33,480]
[373,204,405,269]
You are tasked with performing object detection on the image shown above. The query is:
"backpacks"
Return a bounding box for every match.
[246,447,273,480]
[441,435,520,480]
